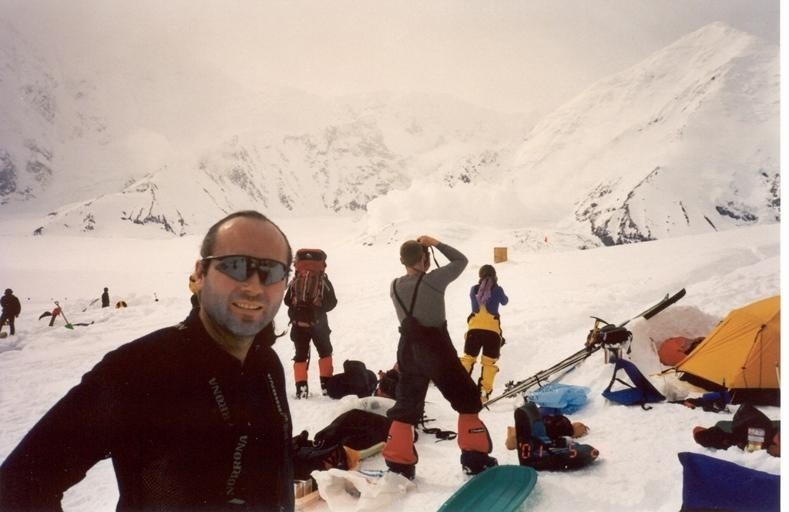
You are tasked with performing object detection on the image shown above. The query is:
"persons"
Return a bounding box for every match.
[101,286,110,308]
[283,262,338,400]
[382,234,500,474]
[1,286,22,338]
[0,210,297,512]
[463,263,509,408]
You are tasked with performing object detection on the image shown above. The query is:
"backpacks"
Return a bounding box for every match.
[291,249,328,328]
[316,410,393,462]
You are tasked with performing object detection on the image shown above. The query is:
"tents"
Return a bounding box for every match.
[676,294,780,404]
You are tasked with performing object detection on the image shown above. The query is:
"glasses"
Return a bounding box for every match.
[203,254,290,285]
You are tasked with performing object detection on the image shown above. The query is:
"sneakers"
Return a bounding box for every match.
[385,460,415,478]
[461,456,496,474]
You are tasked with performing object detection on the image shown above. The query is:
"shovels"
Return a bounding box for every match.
[54,300,74,329]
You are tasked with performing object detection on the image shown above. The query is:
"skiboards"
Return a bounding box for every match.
[482,288,687,409]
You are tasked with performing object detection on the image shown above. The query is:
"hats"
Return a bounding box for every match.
[479,265,495,277]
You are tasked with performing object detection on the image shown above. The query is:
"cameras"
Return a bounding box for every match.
[417,238,429,252]
